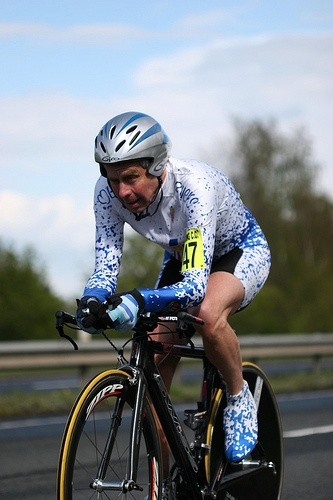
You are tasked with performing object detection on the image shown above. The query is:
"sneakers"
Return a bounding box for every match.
[223,378,259,463]
[145,480,177,500]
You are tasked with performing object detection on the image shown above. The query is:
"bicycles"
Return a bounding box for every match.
[55,310,284,500]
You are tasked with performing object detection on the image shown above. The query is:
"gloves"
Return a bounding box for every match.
[97,288,147,332]
[76,295,106,335]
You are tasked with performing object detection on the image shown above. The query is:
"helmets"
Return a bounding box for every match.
[94,112,170,180]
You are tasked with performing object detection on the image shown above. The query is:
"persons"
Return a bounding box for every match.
[75,112,271,500]
